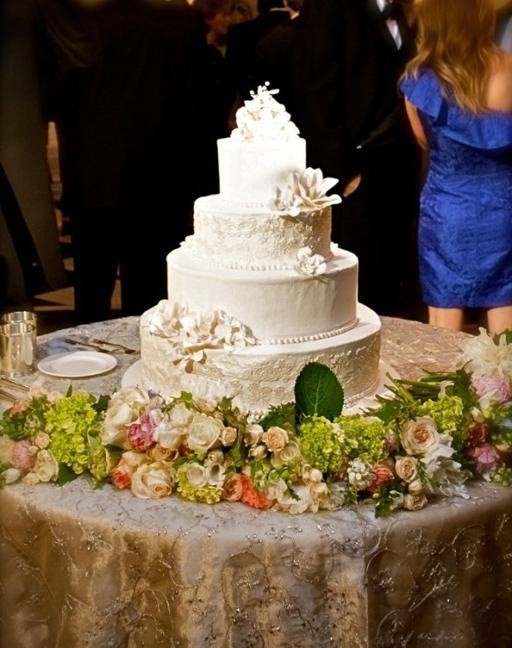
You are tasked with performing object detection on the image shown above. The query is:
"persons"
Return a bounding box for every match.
[1,0,512,343]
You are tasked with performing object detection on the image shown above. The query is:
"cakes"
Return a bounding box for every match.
[140,84,383,428]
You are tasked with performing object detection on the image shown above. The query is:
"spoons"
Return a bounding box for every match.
[93,339,137,354]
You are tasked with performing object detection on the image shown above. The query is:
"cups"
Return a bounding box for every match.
[0,309,38,375]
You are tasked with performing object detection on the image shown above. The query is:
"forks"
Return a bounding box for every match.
[63,338,120,354]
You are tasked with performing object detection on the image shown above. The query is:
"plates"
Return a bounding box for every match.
[37,350,118,378]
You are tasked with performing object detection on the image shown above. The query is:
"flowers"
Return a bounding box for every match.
[0,326,509,519]
